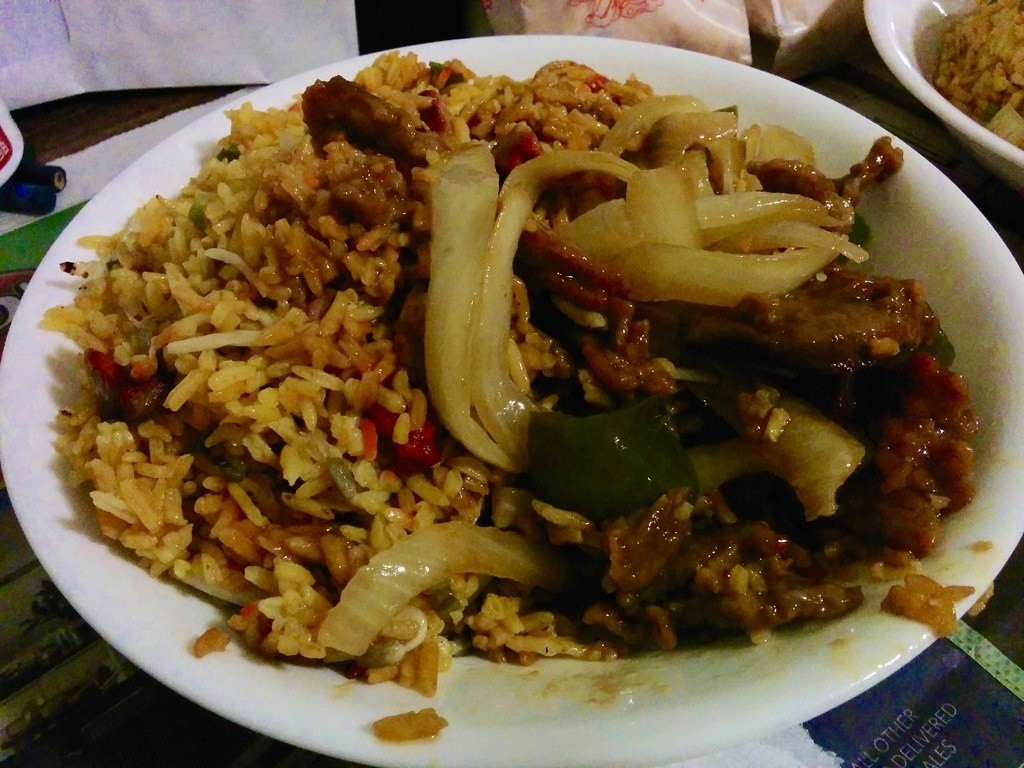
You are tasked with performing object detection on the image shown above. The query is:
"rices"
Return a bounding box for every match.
[34,48,974,740]
[931,2,1024,151]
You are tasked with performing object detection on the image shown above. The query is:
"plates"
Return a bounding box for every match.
[856,0,1024,199]
[0,30,1024,768]
